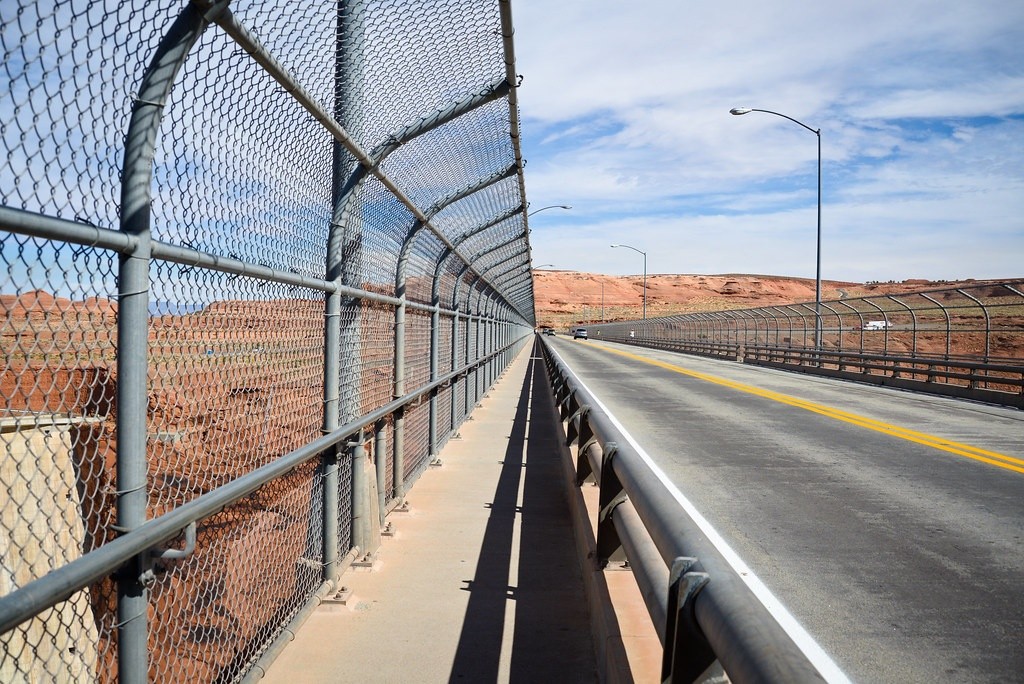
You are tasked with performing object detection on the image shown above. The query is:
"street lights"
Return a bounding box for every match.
[729,105,821,370]
[611,244,646,339]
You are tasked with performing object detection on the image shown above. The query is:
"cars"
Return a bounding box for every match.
[548,329,556,337]
[543,328,549,335]
[574,327,588,340]
